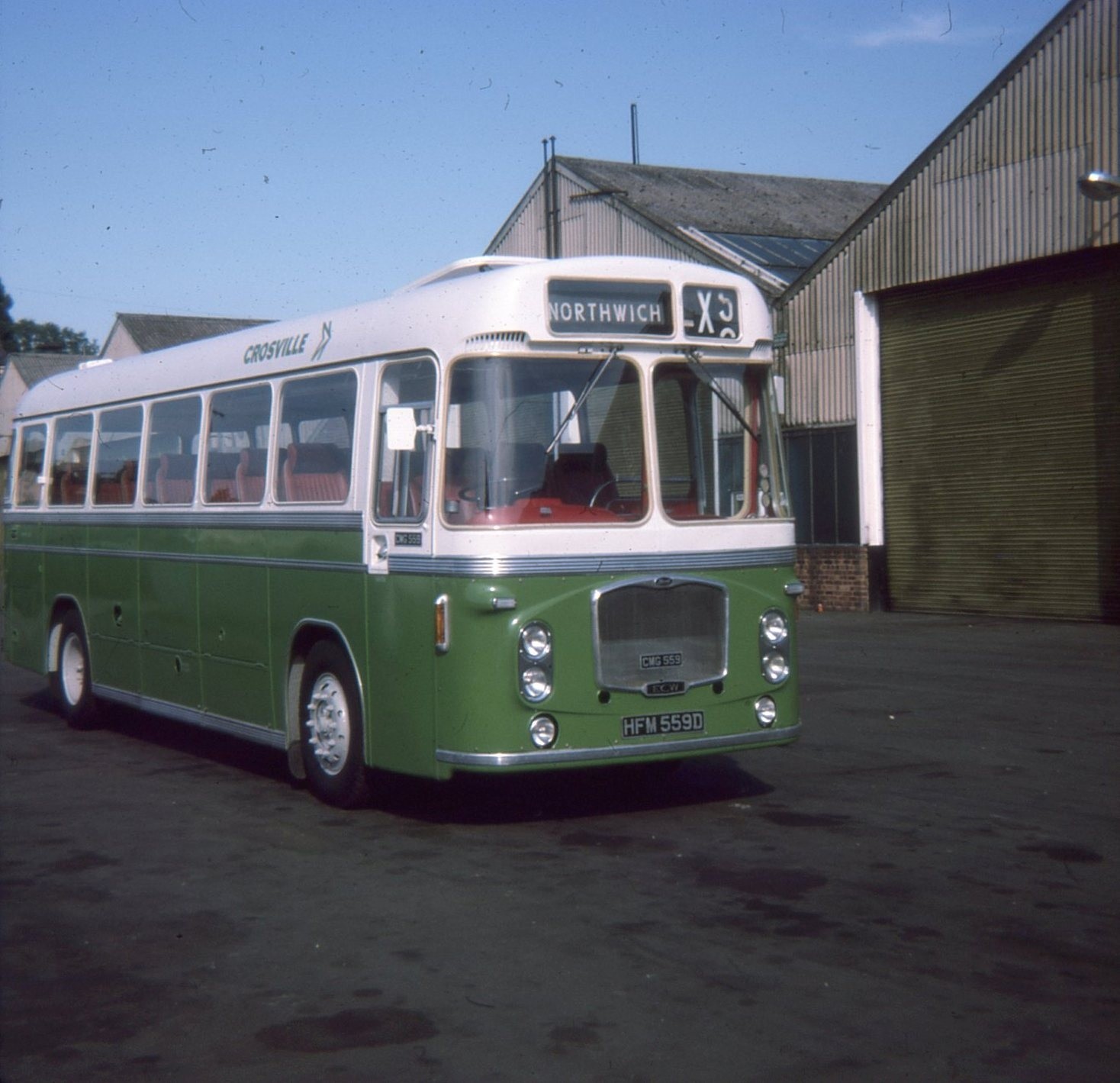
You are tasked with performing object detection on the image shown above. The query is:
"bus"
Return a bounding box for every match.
[0,254,805,810]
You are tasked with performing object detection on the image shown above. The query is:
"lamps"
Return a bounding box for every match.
[1077,171,1120,202]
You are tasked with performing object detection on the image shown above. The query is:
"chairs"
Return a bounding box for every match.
[52,444,618,510]
